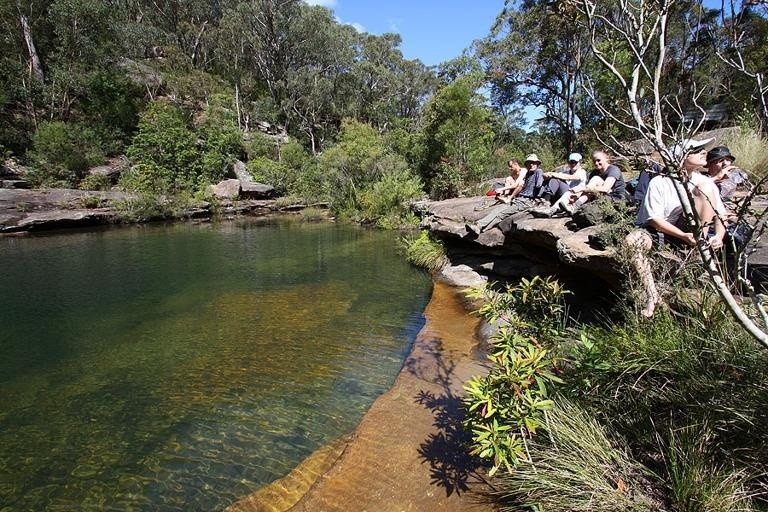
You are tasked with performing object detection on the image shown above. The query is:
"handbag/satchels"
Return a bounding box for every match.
[723,224,750,252]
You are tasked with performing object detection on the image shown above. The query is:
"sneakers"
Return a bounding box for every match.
[466,224,481,235]
[529,207,554,217]
[559,201,581,216]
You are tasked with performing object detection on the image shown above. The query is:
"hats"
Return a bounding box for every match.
[672,136,715,160]
[525,154,542,165]
[569,153,581,161]
[704,147,736,167]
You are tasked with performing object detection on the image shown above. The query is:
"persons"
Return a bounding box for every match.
[628,135,727,318]
[533,152,588,209]
[702,146,750,293]
[623,144,662,215]
[531,149,624,218]
[465,153,544,237]
[494,159,528,203]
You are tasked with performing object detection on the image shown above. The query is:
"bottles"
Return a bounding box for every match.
[724,166,748,185]
